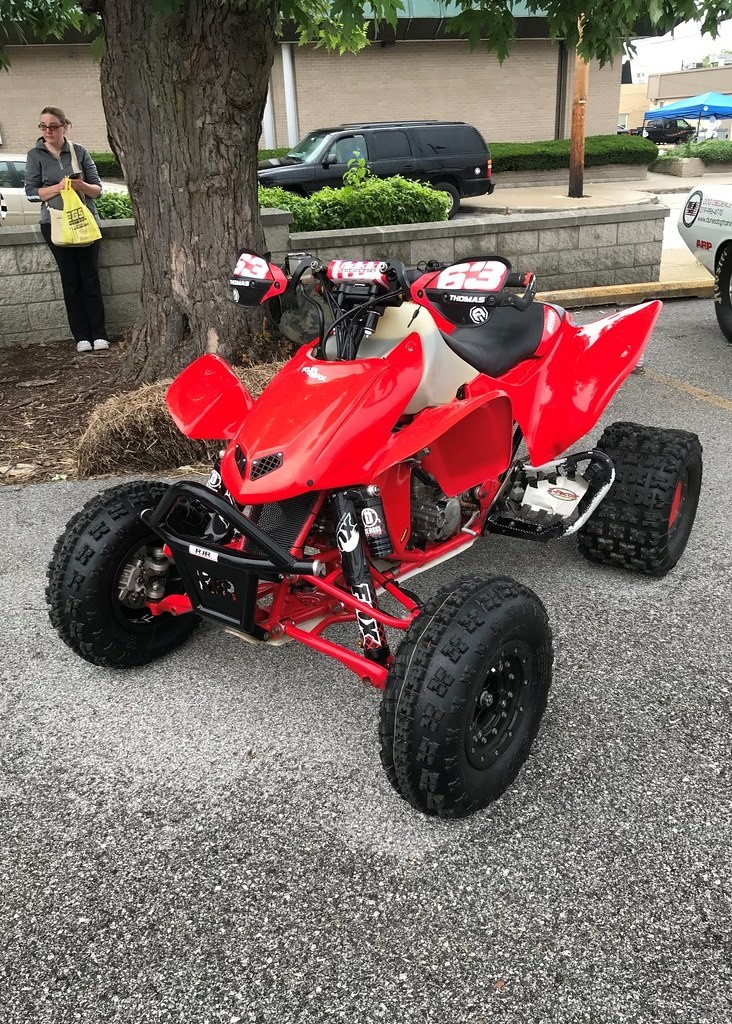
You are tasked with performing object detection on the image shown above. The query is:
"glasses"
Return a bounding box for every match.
[38,122,64,132]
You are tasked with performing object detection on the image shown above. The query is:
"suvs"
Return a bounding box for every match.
[258,120,497,221]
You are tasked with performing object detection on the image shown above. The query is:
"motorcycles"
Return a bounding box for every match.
[46,247,706,821]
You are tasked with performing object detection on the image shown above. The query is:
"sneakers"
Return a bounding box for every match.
[77,340,92,352]
[94,339,110,350]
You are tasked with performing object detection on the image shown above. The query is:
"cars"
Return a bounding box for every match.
[1,152,127,225]
[677,183,732,343]
[619,117,697,144]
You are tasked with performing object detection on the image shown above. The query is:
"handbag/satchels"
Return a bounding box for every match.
[60,178,102,245]
[47,206,94,247]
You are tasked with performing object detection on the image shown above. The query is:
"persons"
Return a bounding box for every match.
[25,106,110,352]
[338,136,364,161]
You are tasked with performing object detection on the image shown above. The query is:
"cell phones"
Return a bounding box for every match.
[69,172,80,179]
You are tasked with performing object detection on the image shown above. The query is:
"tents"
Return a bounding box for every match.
[643,92,732,143]
